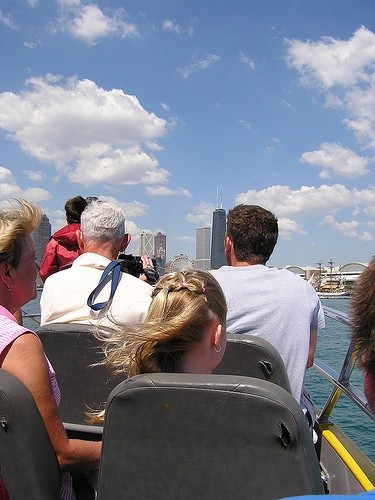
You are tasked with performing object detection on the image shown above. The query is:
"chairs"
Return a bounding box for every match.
[0,323,326,500]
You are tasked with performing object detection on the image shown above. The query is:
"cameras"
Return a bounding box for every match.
[118,254,159,285]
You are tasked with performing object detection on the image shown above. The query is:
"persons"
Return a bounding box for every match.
[1,201,105,499]
[204,206,325,424]
[40,197,89,282]
[36,201,156,326]
[86,270,228,426]
[353,257,375,416]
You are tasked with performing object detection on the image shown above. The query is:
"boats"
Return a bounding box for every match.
[316,288,351,296]
